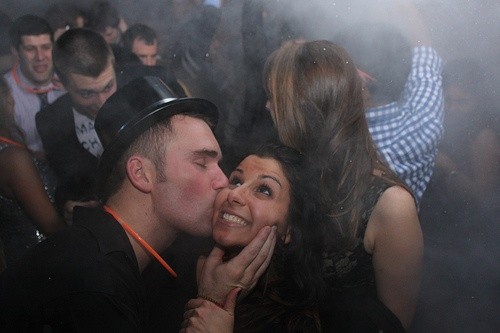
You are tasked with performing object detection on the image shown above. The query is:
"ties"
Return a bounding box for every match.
[37,91,49,110]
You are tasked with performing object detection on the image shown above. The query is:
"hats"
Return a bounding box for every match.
[95,75,218,174]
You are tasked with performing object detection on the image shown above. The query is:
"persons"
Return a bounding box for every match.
[47,0,279,144]
[0,75,70,252]
[0,74,279,333]
[333,0,446,227]
[263,38,423,333]
[0,13,15,75]
[437,56,500,333]
[0,15,69,282]
[34,27,189,225]
[180,141,325,333]
